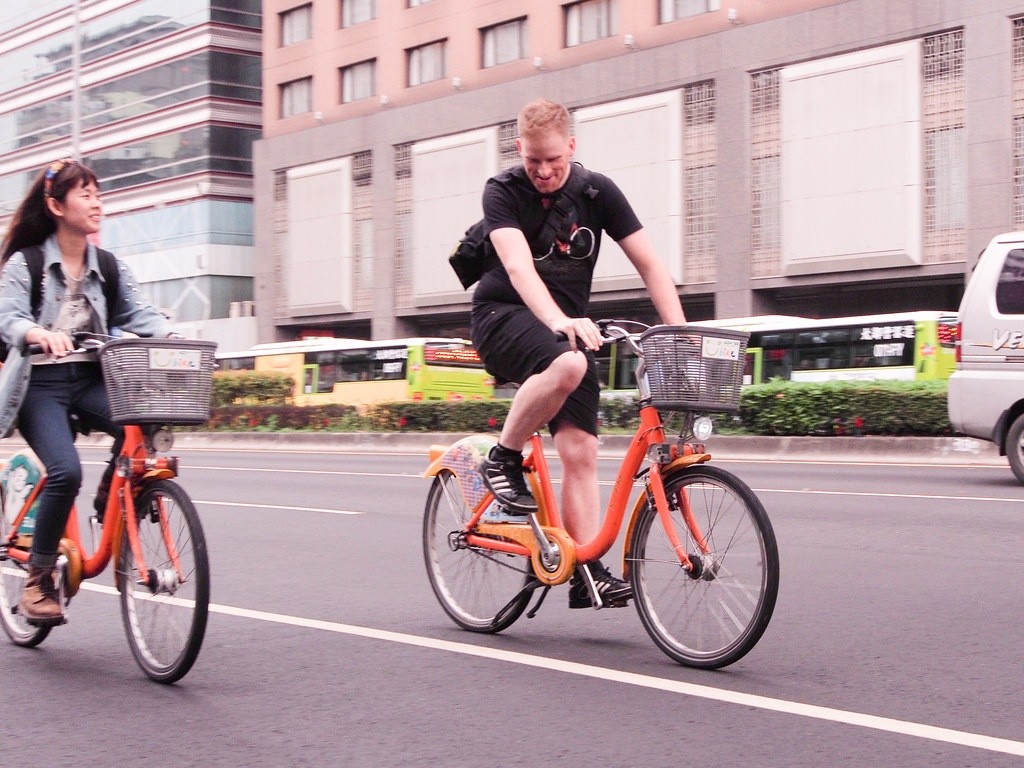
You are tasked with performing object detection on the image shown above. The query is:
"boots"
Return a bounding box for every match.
[18,565,65,626]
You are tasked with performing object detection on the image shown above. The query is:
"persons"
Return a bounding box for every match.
[448,100,689,610]
[0,156,182,627]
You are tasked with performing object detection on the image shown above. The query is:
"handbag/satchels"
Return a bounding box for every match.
[449,164,589,292]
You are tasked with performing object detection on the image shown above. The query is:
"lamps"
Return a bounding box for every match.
[380,96,392,108]
[452,77,464,90]
[624,34,637,50]
[728,8,741,25]
[314,111,323,122]
[533,57,545,71]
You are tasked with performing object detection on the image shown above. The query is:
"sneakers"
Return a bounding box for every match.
[567,563,634,610]
[478,445,539,514]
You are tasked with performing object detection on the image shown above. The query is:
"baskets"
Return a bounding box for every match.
[640,324,751,413]
[97,337,218,427]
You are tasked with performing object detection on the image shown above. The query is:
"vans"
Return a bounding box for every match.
[948,230,1023,485]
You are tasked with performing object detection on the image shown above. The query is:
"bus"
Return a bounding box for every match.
[648,309,957,391]
[214,337,494,416]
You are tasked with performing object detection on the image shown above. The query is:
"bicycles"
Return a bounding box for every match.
[422,317,780,670]
[0,331,219,686]
